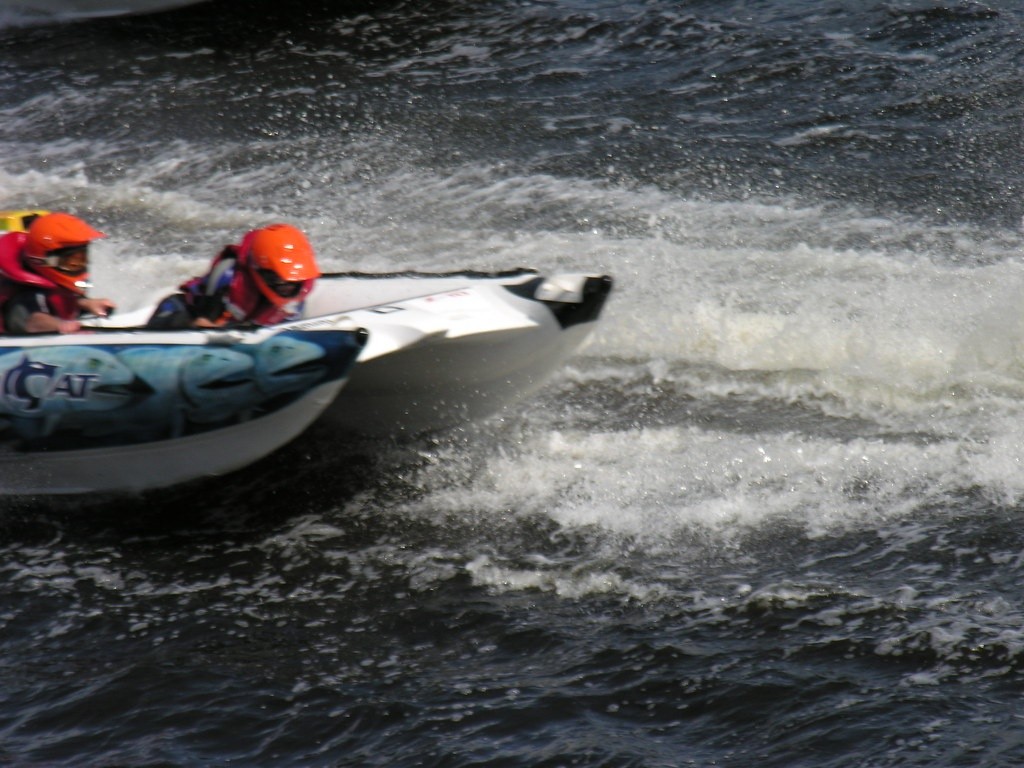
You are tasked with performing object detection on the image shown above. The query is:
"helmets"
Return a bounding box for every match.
[22,214,107,295]
[238,225,321,316]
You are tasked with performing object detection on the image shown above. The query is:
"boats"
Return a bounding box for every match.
[70,260,615,442]
[1,327,369,505]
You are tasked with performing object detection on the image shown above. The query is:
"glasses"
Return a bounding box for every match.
[57,248,89,272]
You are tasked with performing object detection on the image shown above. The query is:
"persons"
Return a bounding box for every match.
[0,210,118,335]
[147,224,320,327]
